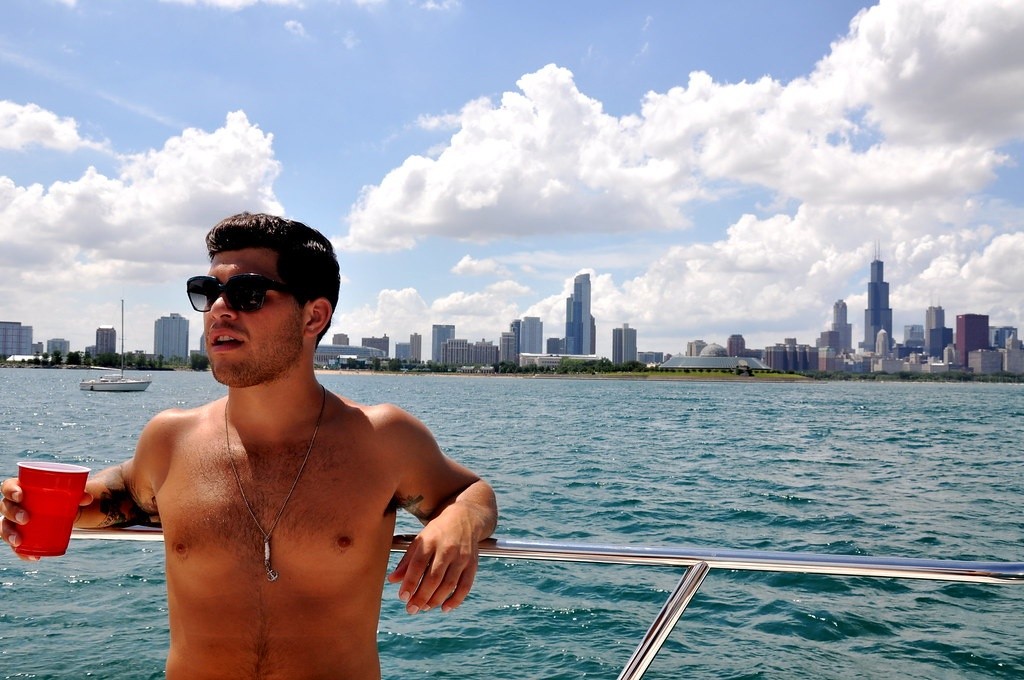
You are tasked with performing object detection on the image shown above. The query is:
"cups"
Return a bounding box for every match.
[14,461,92,556]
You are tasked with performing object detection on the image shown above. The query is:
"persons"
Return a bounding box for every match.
[0,212,498,680]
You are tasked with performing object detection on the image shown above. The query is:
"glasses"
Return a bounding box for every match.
[187,273,311,314]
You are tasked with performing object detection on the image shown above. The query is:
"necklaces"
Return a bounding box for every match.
[225,384,326,581]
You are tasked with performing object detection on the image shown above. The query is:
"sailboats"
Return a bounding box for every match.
[78,297,153,392]
